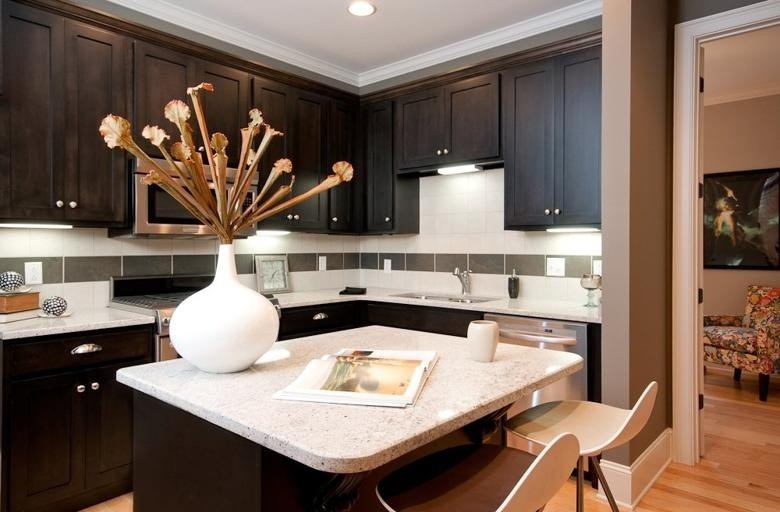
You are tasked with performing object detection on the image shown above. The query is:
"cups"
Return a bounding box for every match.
[467,320,499,362]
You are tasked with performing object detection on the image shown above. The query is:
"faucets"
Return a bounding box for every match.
[453,267,472,294]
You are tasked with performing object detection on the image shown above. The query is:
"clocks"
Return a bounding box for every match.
[255,255,292,294]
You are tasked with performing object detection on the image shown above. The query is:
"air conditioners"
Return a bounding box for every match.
[348,1,375,17]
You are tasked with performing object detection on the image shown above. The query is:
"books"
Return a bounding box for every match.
[273,347,442,409]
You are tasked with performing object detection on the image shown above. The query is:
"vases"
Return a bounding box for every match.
[168,244,279,374]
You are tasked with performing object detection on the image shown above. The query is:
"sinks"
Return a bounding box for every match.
[390,293,499,304]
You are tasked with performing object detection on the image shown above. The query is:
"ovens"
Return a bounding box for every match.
[155,335,182,362]
[483,314,589,472]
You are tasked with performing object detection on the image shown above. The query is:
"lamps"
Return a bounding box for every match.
[438,165,483,175]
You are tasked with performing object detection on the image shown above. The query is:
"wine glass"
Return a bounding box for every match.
[580,273,601,307]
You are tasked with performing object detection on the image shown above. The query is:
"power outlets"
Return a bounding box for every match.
[25,262,43,285]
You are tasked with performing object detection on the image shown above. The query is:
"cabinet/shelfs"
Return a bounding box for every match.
[1,324,156,512]
[360,298,483,338]
[278,299,361,341]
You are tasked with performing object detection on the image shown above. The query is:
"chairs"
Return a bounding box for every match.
[375,432,579,512]
[703,284,780,401]
[504,381,658,512]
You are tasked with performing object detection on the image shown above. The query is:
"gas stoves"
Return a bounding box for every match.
[109,291,282,337]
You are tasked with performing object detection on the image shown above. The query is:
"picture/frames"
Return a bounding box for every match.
[703,167,780,270]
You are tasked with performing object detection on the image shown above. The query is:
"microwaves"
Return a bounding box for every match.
[107,158,259,239]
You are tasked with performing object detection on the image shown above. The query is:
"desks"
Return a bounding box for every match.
[116,325,584,512]
